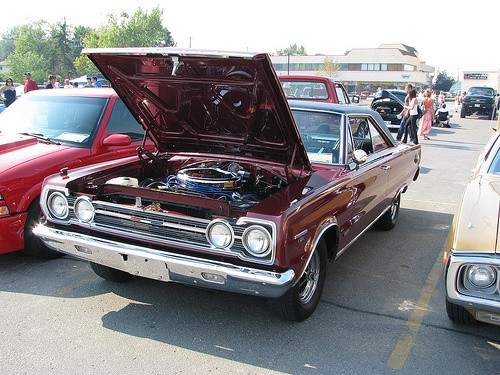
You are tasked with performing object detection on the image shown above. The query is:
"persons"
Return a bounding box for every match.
[0,78,16,110]
[417,91,435,141]
[83,78,93,88]
[454,93,466,112]
[419,88,445,107]
[24,72,39,94]
[436,102,453,128]
[73,82,78,88]
[92,76,102,88]
[497,96,500,110]
[396,83,419,145]
[45,75,59,89]
[282,82,293,98]
[64,79,73,88]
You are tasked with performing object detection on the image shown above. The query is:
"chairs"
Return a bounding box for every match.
[437,111,449,127]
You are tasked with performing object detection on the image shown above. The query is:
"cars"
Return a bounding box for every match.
[370,88,437,126]
[33,47,422,323]
[442,128,500,327]
[351,90,369,103]
[0,87,157,258]
[0,82,46,106]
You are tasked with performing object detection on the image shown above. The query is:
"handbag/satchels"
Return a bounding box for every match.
[417,106,422,119]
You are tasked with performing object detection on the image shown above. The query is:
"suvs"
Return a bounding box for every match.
[247,75,361,116]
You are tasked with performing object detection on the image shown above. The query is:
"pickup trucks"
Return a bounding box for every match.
[460,86,500,120]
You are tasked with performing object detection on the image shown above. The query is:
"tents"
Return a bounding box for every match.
[69,73,111,88]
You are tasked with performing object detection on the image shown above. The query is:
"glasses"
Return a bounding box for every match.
[7,81,12,83]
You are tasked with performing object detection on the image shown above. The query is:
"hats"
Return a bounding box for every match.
[24,72,31,76]
[49,75,56,80]
